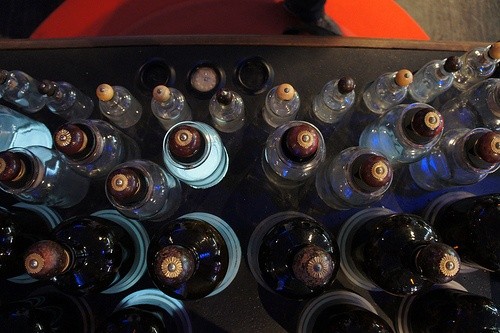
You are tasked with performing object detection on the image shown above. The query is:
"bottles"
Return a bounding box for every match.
[0,41,500,333]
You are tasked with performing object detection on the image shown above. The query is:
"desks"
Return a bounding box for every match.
[0,34,499,332]
